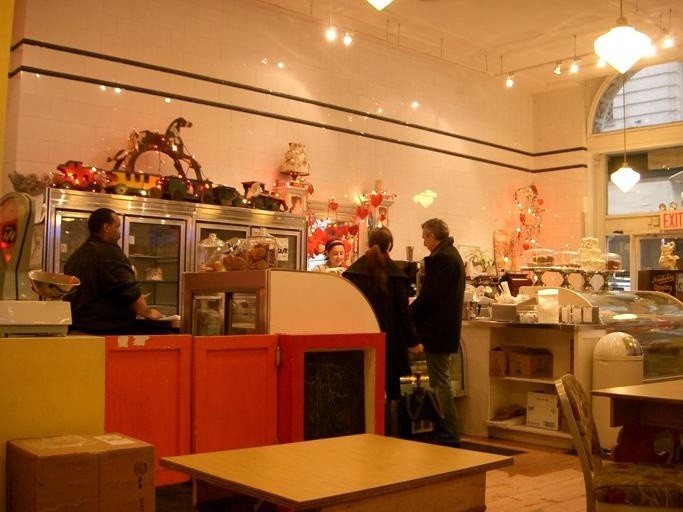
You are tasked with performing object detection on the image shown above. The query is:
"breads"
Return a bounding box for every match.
[532,255,553,262]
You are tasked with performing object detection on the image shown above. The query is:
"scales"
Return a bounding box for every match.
[0,270,81,337]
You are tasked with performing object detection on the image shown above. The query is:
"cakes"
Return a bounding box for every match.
[144,267,162,281]
[579,236,604,271]
[605,261,620,270]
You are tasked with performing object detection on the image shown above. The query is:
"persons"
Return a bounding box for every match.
[311,238,350,276]
[63,207,164,335]
[342,225,413,436]
[410,218,466,446]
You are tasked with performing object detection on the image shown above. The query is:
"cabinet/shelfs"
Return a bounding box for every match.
[486,326,606,452]
[519,286,683,323]
[39,187,307,318]
[638,270,683,303]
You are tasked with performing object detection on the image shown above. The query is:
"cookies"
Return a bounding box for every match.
[205,242,275,272]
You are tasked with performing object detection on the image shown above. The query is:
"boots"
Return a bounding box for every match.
[390,396,407,437]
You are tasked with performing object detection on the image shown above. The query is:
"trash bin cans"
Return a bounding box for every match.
[592,331,644,450]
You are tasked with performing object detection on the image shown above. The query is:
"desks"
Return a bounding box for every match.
[159,434,515,512]
[590,377,682,465]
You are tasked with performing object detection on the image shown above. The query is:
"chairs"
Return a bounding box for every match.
[553,373,683,512]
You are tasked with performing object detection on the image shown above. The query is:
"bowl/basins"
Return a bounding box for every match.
[26,269,81,297]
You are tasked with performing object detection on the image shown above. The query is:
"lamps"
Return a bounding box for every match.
[594,1,652,75]
[610,73,641,192]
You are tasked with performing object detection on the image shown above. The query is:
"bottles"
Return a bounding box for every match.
[222,237,247,271]
[196,232,226,271]
[246,227,279,270]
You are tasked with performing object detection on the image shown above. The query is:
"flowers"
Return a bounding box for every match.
[307,194,386,269]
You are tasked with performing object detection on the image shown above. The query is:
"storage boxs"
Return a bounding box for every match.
[490,345,562,432]
[6,433,155,512]
